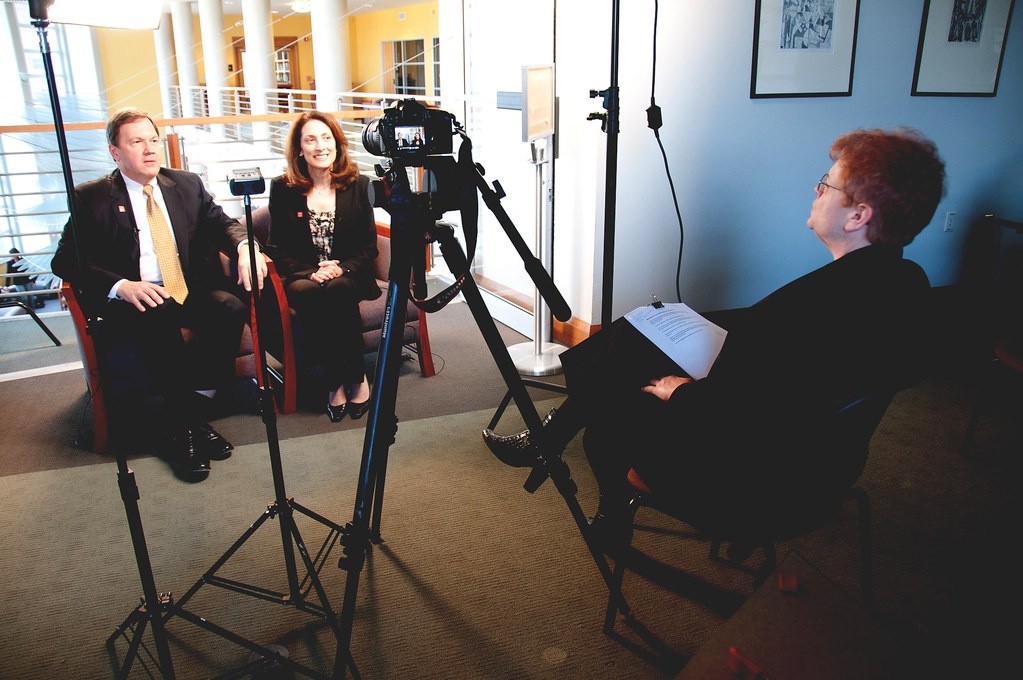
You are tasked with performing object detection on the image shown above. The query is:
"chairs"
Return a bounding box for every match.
[603,209,1023,636]
[61,177,436,448]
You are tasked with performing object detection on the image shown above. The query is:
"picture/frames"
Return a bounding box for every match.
[750,0,861,99]
[910,0,1016,97]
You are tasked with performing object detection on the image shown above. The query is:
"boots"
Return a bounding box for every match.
[586,482,632,545]
[482,407,578,494]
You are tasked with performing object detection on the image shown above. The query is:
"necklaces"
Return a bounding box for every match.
[312,183,333,195]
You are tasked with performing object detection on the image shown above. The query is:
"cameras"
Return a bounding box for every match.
[362,99,452,157]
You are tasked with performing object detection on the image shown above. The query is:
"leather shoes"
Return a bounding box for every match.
[349,373,371,418]
[326,382,348,422]
[174,423,210,482]
[199,421,233,460]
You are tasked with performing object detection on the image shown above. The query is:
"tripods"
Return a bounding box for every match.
[31,22,631,680]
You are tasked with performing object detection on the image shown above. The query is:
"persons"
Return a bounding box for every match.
[396,130,424,147]
[407,72,416,94]
[269,111,383,423]
[483,127,948,554]
[50,108,268,474]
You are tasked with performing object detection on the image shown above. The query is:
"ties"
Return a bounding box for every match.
[142,184,190,305]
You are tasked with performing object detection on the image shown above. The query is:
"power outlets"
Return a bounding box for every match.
[944,212,956,232]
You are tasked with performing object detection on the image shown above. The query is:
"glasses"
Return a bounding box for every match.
[817,173,847,197]
